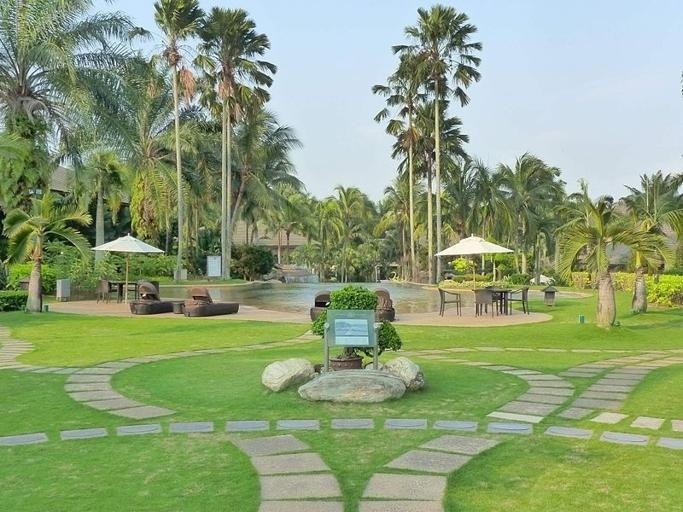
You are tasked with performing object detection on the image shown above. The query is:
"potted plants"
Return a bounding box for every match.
[312,285,403,373]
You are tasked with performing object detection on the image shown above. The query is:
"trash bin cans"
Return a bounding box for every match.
[56,279,71,302]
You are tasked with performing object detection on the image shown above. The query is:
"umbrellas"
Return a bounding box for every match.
[433,233,514,306]
[89,233,165,304]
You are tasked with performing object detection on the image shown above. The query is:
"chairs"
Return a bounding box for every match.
[310,290,331,323]
[438,288,462,317]
[183,287,239,317]
[96,280,112,304]
[130,283,185,315]
[372,287,395,322]
[508,287,529,315]
[151,281,160,296]
[485,287,503,314]
[475,289,493,317]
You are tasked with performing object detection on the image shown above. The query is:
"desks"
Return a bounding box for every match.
[107,281,141,304]
[472,288,512,315]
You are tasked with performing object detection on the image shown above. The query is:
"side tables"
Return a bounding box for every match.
[172,302,184,313]
